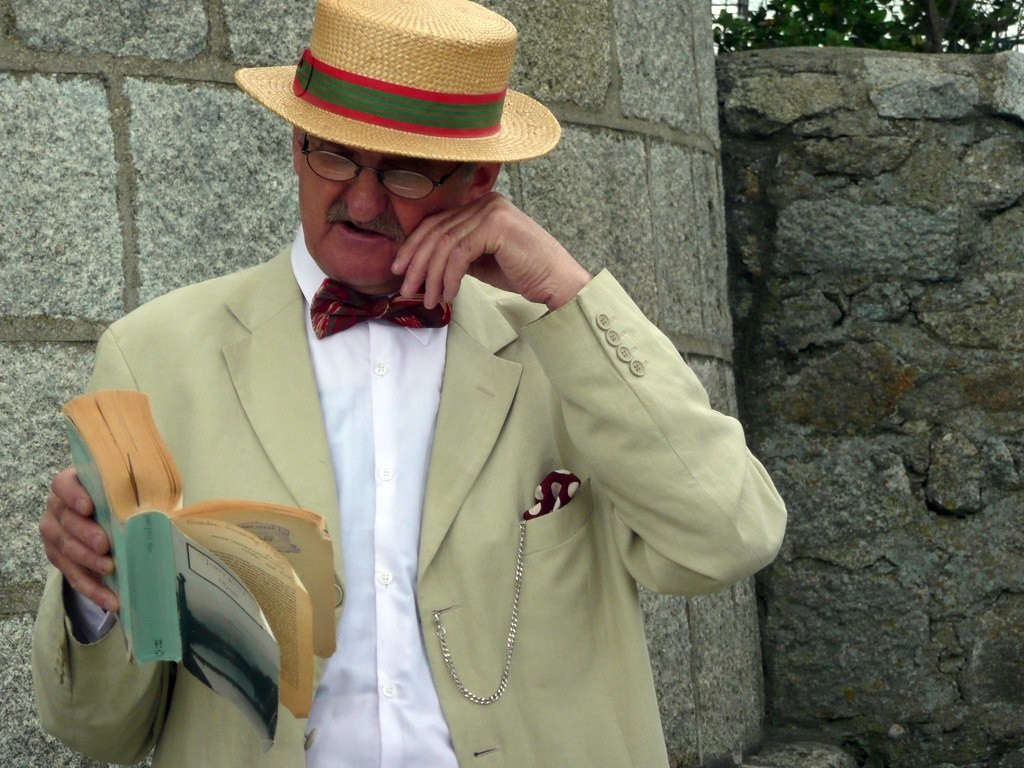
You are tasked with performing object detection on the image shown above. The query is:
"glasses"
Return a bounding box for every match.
[301,130,463,200]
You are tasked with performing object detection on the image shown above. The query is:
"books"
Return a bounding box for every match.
[64,390,336,751]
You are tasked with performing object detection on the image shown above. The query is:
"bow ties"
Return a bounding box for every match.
[309,278,452,340]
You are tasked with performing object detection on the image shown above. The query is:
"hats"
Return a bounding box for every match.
[232,0,562,164]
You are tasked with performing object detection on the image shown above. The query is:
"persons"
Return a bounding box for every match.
[31,0,789,768]
